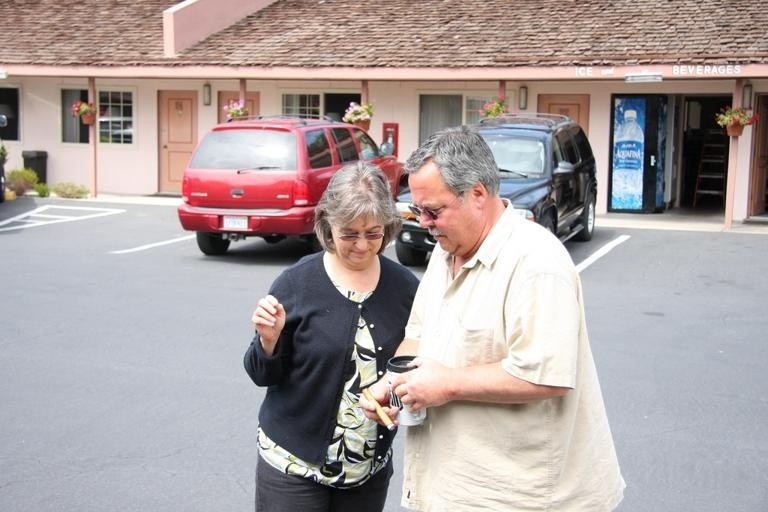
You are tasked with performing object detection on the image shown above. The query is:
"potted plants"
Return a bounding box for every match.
[3,169,39,198]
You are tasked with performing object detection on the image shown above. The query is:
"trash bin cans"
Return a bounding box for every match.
[22,150,47,186]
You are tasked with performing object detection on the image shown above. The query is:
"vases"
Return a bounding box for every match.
[355,120,371,130]
[82,111,99,125]
[725,125,745,137]
[234,111,250,120]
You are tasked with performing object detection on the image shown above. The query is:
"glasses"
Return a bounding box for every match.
[331,224,388,241]
[407,193,461,220]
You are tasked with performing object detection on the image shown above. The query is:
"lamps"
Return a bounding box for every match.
[517,85,527,110]
[741,83,753,111]
[202,82,212,107]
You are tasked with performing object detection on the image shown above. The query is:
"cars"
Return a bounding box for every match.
[1,114,8,202]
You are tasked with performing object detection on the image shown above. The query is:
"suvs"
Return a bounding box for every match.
[177,113,406,255]
[392,112,598,266]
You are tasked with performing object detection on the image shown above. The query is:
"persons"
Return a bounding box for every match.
[240,163,422,510]
[358,124,630,512]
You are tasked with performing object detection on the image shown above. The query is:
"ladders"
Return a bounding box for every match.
[692,126,728,215]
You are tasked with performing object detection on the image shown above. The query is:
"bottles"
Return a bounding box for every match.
[384,354,429,429]
[612,109,644,196]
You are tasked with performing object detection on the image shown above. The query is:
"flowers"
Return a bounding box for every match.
[714,105,761,127]
[222,100,250,120]
[480,97,509,118]
[341,102,374,122]
[70,98,98,116]
